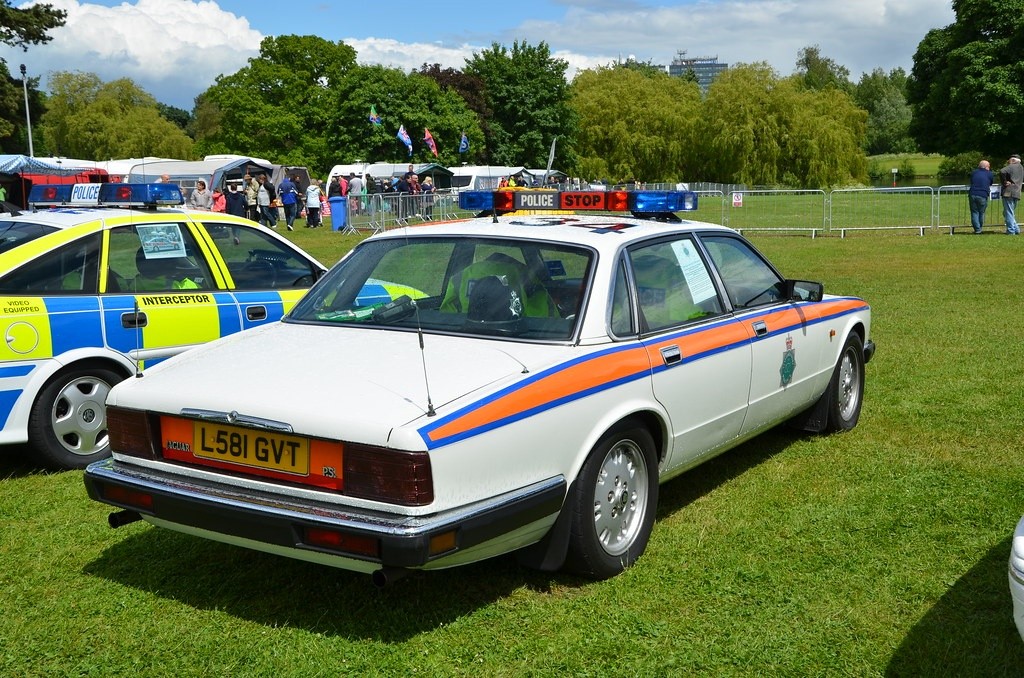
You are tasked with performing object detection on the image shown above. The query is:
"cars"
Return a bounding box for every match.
[0,183,432,467]
[83,188,875,578]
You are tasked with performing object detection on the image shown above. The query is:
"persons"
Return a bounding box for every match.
[161,167,436,231]
[999,154,1023,236]
[968,160,993,235]
[496,175,645,191]
[0,184,7,202]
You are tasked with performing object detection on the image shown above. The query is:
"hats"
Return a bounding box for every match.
[244,174,253,180]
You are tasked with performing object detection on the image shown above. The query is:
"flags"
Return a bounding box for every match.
[397,124,413,156]
[422,128,438,158]
[369,105,383,128]
[458,131,471,152]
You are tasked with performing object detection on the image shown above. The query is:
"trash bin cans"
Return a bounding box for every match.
[327,196,348,231]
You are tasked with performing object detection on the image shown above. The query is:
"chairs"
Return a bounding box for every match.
[134,241,200,282]
[456,254,537,318]
[633,255,696,321]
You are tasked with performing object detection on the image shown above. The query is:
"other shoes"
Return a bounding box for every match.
[234,237,239,245]
[287,225,293,230]
[271,222,277,228]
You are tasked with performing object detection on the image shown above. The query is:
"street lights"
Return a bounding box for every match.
[19,64,35,155]
